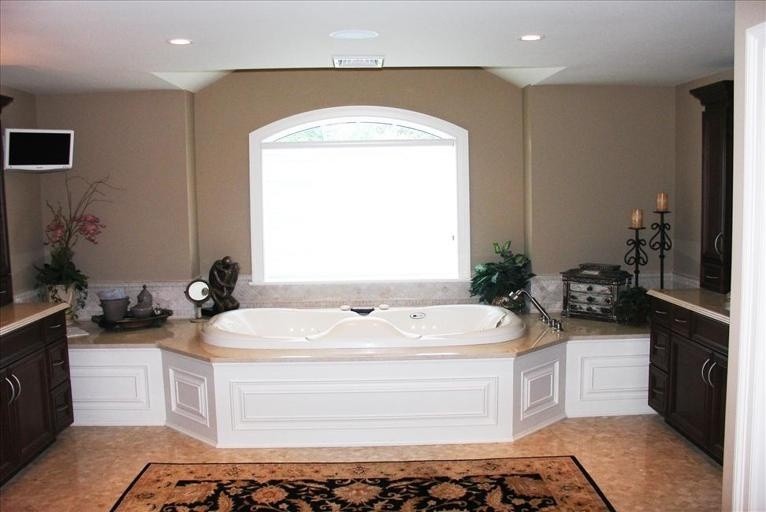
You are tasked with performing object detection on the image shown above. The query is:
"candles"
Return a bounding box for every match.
[631,208,643,228]
[657,192,668,211]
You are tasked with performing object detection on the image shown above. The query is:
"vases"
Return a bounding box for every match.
[48,284,89,337]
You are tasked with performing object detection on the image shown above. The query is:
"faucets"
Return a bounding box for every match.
[509,289,554,326]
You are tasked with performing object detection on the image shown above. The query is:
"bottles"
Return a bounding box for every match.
[137,285,152,305]
[154,304,163,316]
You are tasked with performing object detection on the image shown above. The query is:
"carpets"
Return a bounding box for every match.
[109,456,616,512]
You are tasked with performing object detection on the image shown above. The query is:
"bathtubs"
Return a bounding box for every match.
[202,302,526,349]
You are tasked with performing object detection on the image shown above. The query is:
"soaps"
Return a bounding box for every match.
[340,305,350,310]
[379,304,388,308]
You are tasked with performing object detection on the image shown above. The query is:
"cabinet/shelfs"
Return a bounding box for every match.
[689,80,734,293]
[0,96,12,305]
[0,311,73,487]
[648,298,729,465]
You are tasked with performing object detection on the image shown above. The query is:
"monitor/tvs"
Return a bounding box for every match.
[3,128,74,170]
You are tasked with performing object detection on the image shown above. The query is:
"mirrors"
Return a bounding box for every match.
[184,280,211,323]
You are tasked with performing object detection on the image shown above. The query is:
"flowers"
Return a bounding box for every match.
[34,173,123,326]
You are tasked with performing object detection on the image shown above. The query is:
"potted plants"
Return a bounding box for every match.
[471,241,535,310]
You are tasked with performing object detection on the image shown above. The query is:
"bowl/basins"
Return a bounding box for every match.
[130,306,152,317]
